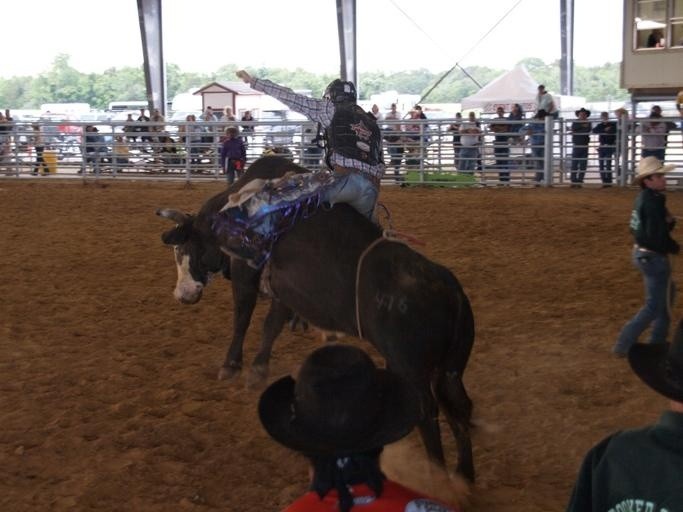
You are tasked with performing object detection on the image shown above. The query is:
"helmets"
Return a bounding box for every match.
[324,79,357,102]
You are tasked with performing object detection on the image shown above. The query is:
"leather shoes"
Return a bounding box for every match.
[221,246,266,269]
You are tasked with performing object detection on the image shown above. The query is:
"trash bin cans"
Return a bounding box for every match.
[39,150,56,175]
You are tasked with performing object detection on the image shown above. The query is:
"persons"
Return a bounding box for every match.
[647,27,664,48]
[610,156,680,358]
[567,319,682,512]
[256,344,461,511]
[217,68,387,272]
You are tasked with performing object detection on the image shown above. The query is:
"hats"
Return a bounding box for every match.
[628,319,682,404]
[256,345,424,451]
[576,108,590,118]
[534,109,550,118]
[225,126,237,136]
[632,155,673,185]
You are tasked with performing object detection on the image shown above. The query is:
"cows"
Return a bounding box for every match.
[155,154,475,486]
